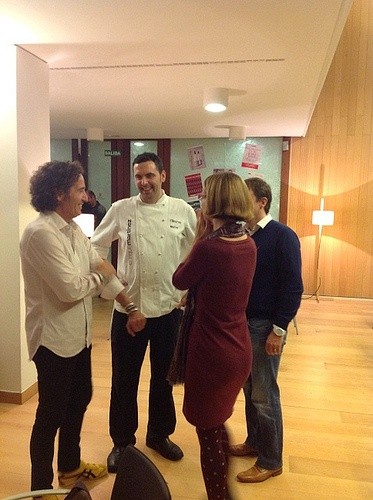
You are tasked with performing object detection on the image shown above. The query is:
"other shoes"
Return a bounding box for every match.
[57,459,107,487]
[34,495,58,500]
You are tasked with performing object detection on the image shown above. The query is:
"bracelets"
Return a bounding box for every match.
[92,270,105,288]
[124,302,139,314]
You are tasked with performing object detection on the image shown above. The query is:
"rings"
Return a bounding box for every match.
[273,349,277,352]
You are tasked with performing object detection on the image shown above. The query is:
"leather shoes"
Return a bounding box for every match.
[230,442,257,457]
[236,463,283,483]
[107,446,124,473]
[146,432,184,461]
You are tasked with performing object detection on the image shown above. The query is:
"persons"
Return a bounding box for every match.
[171,173,257,500]
[92,152,197,474]
[229,177,304,482]
[19,160,146,500]
[82,189,106,231]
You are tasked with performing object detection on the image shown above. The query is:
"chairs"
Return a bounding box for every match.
[5,445,171,500]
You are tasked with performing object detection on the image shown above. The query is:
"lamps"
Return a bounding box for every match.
[72,213,95,238]
[204,87,229,112]
[300,198,335,303]
[229,126,247,140]
[87,129,103,142]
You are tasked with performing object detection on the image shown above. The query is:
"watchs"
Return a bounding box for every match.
[273,327,286,337]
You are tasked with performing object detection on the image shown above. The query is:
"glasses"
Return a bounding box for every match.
[197,193,207,200]
